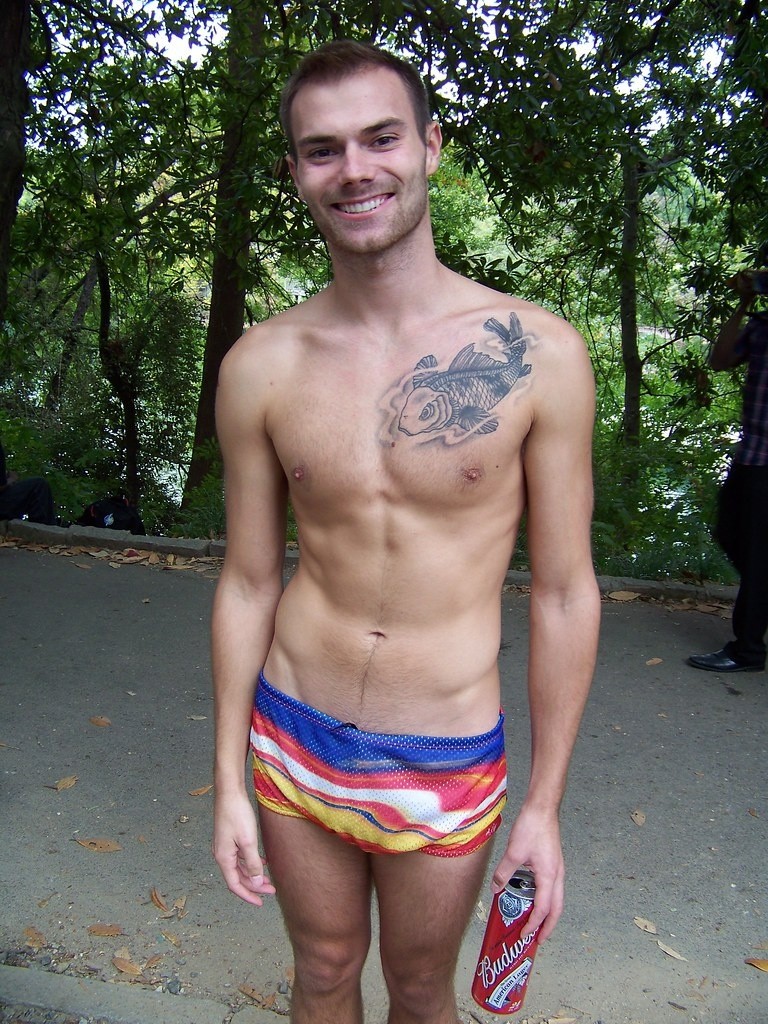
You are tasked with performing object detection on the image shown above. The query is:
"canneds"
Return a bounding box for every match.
[470,870,547,1015]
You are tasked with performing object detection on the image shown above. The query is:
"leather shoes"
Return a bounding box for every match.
[688,648,767,672]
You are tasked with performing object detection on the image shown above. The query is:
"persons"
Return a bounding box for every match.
[684,269,768,671]
[213,38,603,1022]
